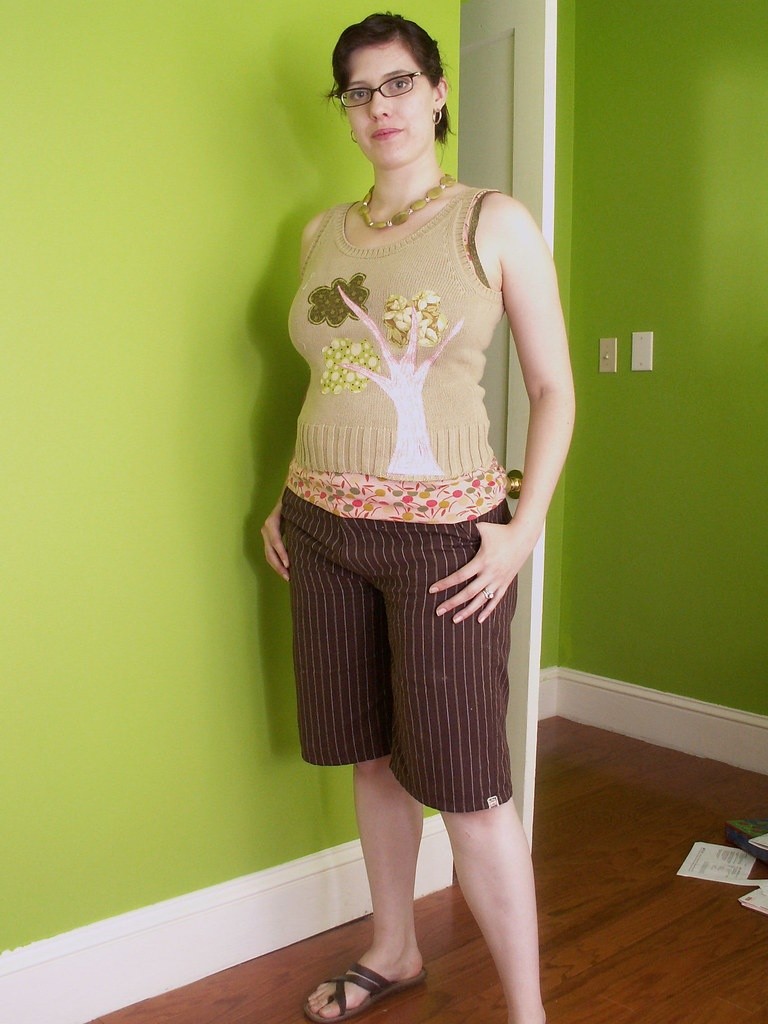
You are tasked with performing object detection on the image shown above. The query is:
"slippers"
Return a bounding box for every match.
[304,963,427,1023]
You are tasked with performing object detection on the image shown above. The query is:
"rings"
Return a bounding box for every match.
[483,589,493,600]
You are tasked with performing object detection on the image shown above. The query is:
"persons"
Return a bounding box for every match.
[260,12,577,1024]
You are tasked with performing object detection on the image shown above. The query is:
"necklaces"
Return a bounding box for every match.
[358,174,458,229]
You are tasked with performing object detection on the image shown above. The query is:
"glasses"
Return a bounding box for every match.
[339,72,427,108]
